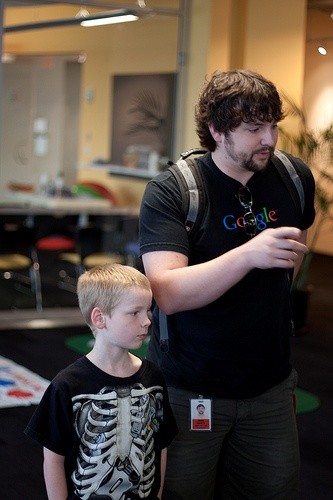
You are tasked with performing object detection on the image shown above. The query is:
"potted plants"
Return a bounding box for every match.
[275,90,333,330]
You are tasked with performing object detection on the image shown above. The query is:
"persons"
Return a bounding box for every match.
[22,263,179,500]
[139,68,317,500]
